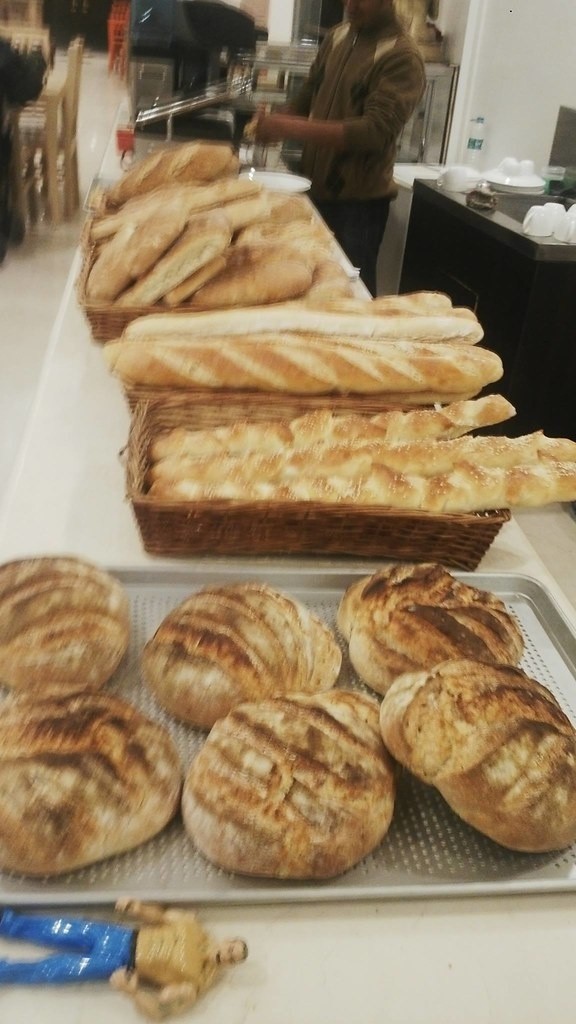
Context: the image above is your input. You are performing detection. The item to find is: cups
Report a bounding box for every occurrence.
[436,168,468,193]
[489,156,542,185]
[522,201,576,242]
[540,164,566,193]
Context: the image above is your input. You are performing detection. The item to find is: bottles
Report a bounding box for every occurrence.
[464,117,486,168]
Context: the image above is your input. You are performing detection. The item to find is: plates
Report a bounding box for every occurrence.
[237,170,312,193]
[484,174,546,196]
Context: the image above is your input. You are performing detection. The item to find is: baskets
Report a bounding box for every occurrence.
[120,385,512,573]
[77,191,303,340]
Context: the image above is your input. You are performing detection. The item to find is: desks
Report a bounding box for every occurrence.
[1,97,574,1024]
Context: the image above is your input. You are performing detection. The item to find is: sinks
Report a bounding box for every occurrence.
[455,190,576,228]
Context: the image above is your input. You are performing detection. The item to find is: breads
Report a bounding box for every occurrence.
[103,291,503,405]
[88,141,356,309]
[146,394,576,513]
[0,557,576,881]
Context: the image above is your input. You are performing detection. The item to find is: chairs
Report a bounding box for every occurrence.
[107,3,129,76]
[0,24,82,222]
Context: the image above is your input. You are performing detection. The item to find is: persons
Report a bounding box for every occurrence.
[248,1,426,301]
[0,35,47,265]
[1,897,249,1023]
